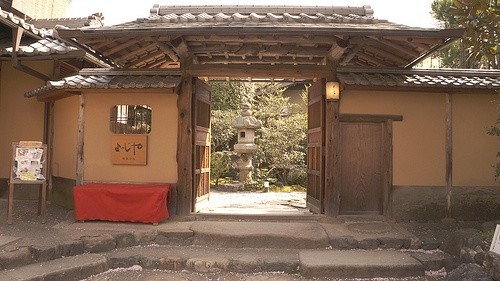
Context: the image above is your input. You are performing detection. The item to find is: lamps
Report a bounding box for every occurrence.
[326,77,340,102]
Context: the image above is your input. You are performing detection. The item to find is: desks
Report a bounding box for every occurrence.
[74,182,174,226]
